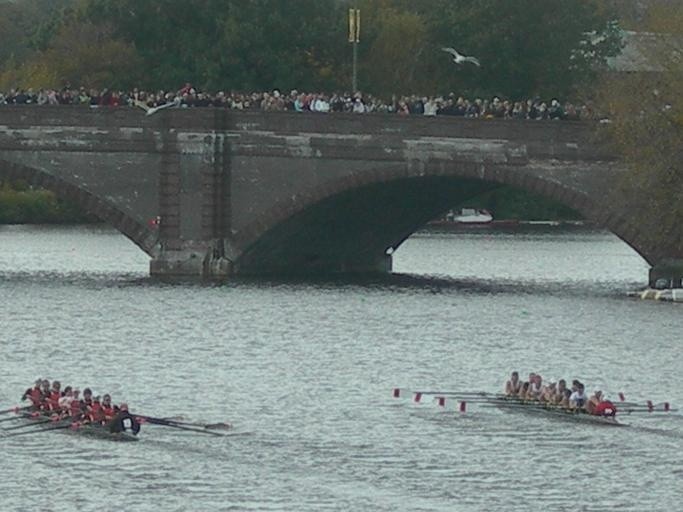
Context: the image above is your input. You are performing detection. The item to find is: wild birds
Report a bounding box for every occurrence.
[442,47,480,66]
[130,98,179,117]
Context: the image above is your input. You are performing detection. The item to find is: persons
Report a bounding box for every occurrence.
[505,371,603,414]
[1,81,600,120]
[22,377,127,433]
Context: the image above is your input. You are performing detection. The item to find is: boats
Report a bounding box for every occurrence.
[13,406,140,442]
[423,205,520,231]
[487,396,632,428]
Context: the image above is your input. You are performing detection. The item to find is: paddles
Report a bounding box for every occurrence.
[603,392,682,419]
[411,391,571,411]
[134,414,231,436]
